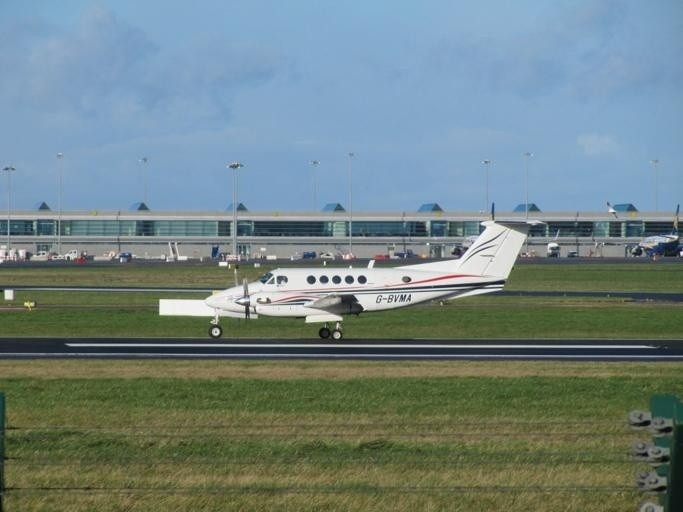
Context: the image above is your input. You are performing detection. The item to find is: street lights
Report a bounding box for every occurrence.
[138,157,150,203]
[649,159,657,211]
[55,151,63,255]
[343,149,354,251]
[481,159,493,211]
[523,150,534,217]
[226,161,244,254]
[308,160,320,212]
[2,165,17,256]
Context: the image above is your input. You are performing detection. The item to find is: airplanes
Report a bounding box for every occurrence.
[603,203,683,259]
[205,220,546,341]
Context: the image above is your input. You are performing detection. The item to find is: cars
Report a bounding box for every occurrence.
[34,249,78,261]
